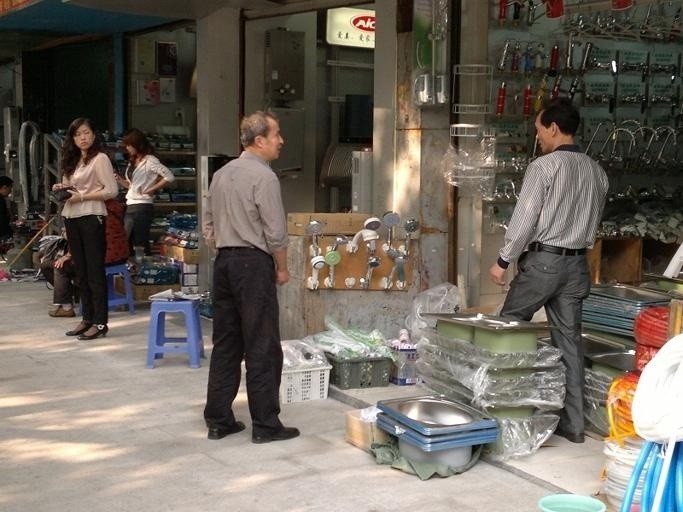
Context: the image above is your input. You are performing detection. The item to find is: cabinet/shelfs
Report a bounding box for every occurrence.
[44,134,198,252]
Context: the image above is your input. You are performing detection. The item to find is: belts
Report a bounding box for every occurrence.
[528,241,586,257]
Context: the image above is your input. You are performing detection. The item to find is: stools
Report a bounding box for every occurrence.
[146,298,204,369]
[79,265,134,316]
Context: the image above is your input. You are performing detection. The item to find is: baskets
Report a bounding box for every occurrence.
[390,348,425,385]
[279,362,333,403]
[326,352,393,389]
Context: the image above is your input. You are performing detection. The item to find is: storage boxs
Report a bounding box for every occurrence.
[345,406,390,454]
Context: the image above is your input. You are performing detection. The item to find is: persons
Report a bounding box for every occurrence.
[202,111,301,442]
[114,128,175,256]
[488,97,609,443]
[52,117,118,340]
[0,175,15,255]
[48,199,129,316]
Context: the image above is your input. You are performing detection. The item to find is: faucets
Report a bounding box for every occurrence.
[496,0,683,171]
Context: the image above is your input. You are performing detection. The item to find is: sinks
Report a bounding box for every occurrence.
[374,281,682,466]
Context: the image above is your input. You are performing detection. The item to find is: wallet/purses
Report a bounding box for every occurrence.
[52,185,77,201]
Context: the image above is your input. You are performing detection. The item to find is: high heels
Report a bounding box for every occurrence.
[66,320,108,340]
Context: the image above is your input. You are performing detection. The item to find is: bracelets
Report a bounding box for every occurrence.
[81,194,84,202]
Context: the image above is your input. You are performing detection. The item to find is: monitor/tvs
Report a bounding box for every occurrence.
[317,7,375,52]
[338,94,372,142]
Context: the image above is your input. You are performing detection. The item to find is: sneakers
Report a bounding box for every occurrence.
[50,305,76,317]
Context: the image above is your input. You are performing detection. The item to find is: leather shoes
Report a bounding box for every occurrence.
[209,422,245,440]
[252,427,299,442]
